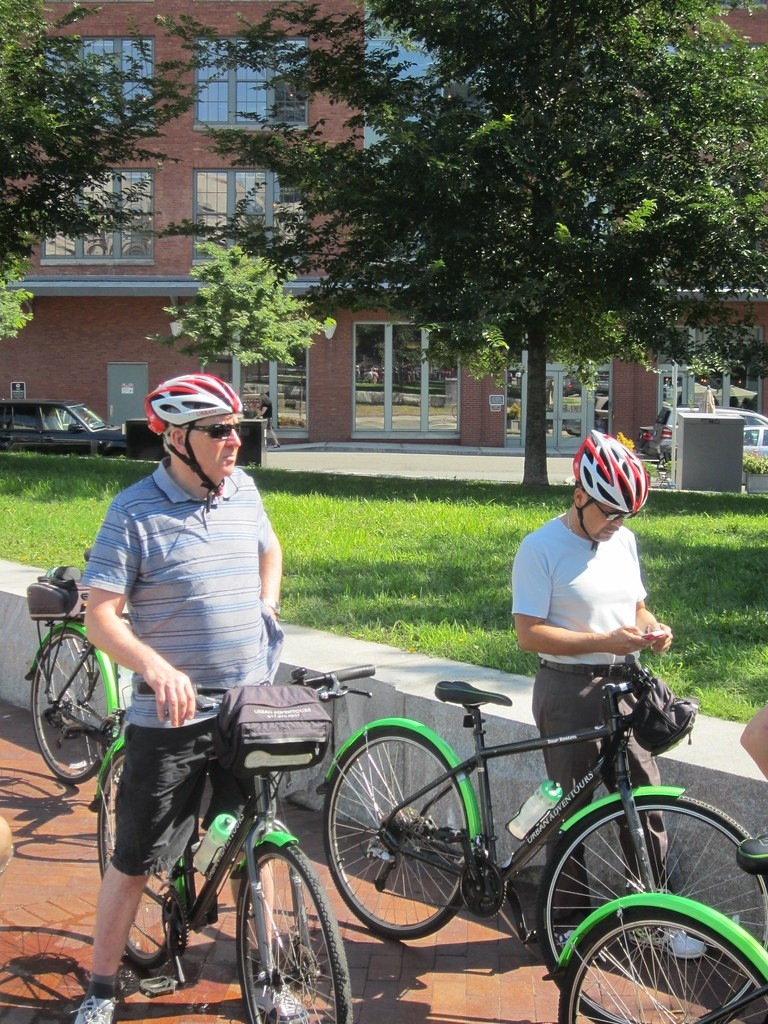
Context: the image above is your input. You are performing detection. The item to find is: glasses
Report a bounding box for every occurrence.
[580,487,638,520]
[179,423,241,440]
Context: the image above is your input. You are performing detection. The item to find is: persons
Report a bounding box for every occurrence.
[71,370,284,1024]
[256,391,281,449]
[740,702,768,781]
[356,363,416,384]
[512,429,708,958]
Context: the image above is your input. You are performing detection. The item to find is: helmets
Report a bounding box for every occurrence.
[573,429,649,514]
[145,374,243,434]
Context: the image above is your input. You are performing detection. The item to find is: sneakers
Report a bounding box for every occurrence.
[253,980,309,1024]
[552,929,597,958]
[628,926,707,958]
[70,997,115,1024]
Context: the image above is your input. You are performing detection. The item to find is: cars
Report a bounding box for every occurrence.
[0,398,127,457]
[562,378,581,397]
[652,403,768,462]
[284,381,306,401]
[566,385,609,436]
[596,374,609,395]
[635,427,656,456]
[743,425,768,455]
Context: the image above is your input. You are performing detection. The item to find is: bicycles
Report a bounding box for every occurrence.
[88,664,376,1024]
[317,654,768,1023]
[542,833,767,1023]
[24,548,125,784]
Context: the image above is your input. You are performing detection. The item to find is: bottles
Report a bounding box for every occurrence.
[194,813,237,872]
[509,779,562,839]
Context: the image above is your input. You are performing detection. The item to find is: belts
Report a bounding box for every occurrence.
[540,659,634,680]
